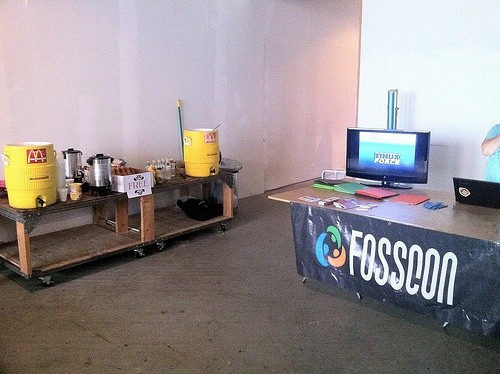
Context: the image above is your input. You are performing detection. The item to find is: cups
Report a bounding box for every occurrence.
[65,179,74,194]
[69,182,82,201]
[58,188,68,201]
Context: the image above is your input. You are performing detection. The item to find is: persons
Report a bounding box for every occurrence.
[481,124,499,183]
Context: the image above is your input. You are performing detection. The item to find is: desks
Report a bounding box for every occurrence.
[267,178,500,340]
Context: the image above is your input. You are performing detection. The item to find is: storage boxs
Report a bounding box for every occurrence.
[111,171,154,193]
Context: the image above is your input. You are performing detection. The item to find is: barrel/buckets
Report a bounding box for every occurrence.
[183,128,219,177]
[87,153,114,195]
[61,148,83,183]
[0,141,57,209]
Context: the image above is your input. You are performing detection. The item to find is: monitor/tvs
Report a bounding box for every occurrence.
[347,127,431,190]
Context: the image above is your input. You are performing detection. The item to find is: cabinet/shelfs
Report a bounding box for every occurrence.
[0,166,239,288]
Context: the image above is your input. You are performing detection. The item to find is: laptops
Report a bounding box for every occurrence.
[452,177,500,210]
